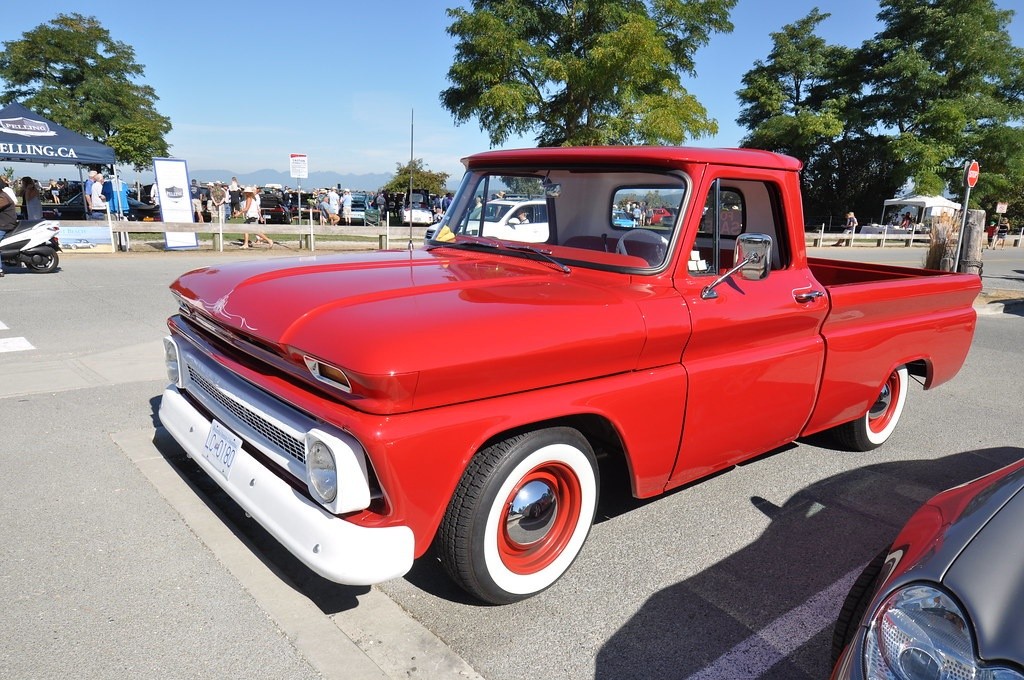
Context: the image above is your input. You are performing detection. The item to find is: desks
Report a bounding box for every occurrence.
[859,225,921,235]
[297,208,322,225]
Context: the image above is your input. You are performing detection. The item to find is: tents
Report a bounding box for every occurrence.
[881,187,961,227]
[0,100,131,251]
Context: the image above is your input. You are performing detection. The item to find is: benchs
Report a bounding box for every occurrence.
[293,215,302,224]
[563,236,737,275]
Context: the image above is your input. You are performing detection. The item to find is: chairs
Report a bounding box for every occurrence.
[364,209,380,227]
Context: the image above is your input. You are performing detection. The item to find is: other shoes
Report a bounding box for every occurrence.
[270,240,273,248]
[239,245,248,249]
[0,272,4,277]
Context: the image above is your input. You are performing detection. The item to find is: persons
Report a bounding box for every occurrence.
[832,211,858,247]
[986,218,1010,250]
[890,212,912,229]
[0,168,672,277]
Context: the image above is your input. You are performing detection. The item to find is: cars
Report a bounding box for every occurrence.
[41,191,161,221]
[646,208,671,225]
[611,211,639,228]
[42,180,370,225]
[424,195,549,246]
[827,458,1024,680]
[398,188,433,226]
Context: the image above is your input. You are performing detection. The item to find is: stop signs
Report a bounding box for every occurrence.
[967,161,979,188]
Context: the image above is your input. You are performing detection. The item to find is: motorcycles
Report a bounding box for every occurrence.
[0,220,62,273]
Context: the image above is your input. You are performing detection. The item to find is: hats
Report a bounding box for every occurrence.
[221,183,228,186]
[192,179,196,182]
[243,187,253,192]
[215,181,221,184]
[331,187,337,191]
[344,189,349,192]
[850,212,854,216]
[206,182,214,186]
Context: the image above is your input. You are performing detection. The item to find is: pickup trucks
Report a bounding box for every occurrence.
[155,146,984,607]
